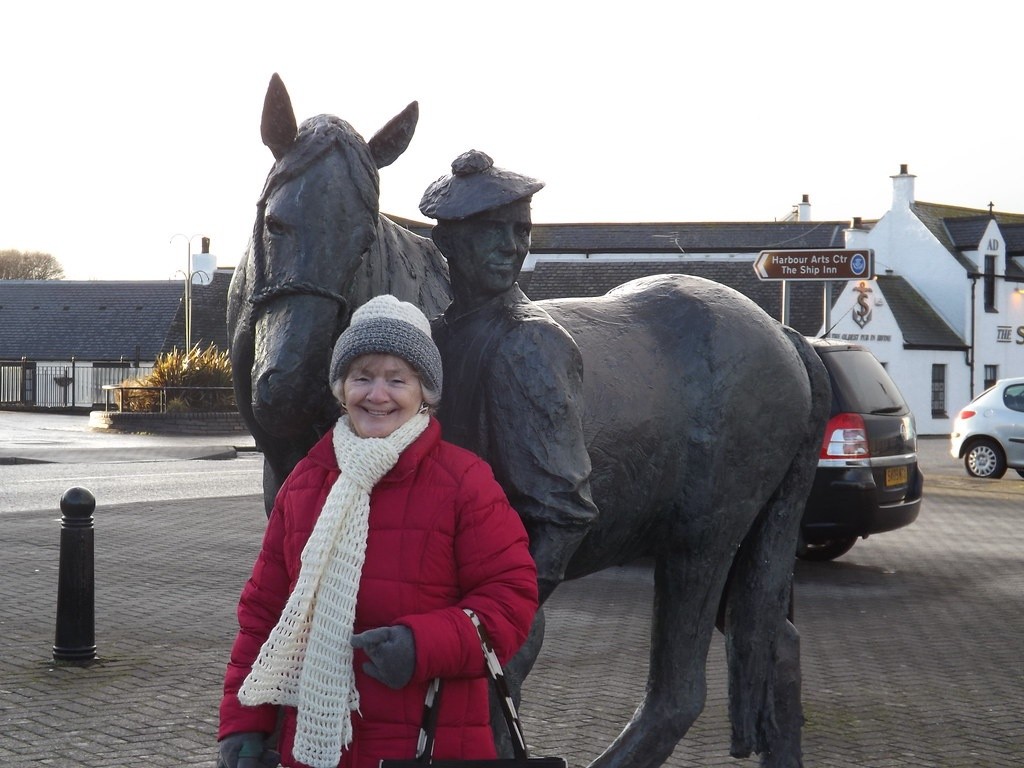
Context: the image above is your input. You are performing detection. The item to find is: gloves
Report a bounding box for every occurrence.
[351,626,415,690]
[217,731,281,768]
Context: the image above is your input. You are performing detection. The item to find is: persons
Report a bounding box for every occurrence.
[216,294,539,768]
[420,149,600,708]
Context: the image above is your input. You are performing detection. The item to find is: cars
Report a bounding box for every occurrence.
[950,377,1024,479]
[795,336,924,560]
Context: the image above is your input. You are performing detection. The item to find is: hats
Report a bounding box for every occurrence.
[329,294,443,396]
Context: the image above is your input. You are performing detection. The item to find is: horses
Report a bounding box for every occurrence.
[227,71,832,768]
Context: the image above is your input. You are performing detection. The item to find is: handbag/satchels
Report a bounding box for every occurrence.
[378,610,568,768]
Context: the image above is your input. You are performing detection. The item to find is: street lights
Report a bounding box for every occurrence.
[168,234,210,363]
